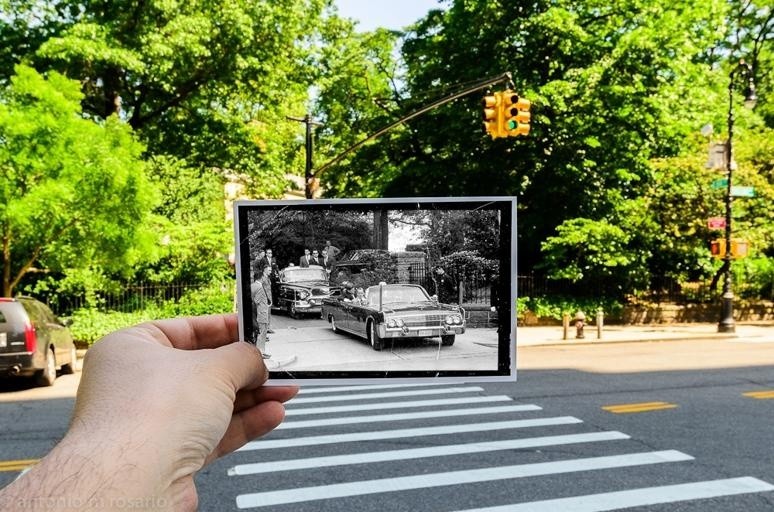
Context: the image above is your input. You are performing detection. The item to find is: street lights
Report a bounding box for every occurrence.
[716,61,753,334]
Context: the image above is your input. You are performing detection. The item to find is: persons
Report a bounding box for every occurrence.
[251,241,387,359]
[0,313,300,512]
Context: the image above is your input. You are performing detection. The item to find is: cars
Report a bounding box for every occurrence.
[319,282,469,351]
[0,294,78,388]
[270,265,342,321]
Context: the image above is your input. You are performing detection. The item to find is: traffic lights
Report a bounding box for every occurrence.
[482,89,531,140]
[730,238,751,260]
[709,237,727,259]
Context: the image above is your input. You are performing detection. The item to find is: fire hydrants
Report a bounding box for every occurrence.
[574,311,588,339]
